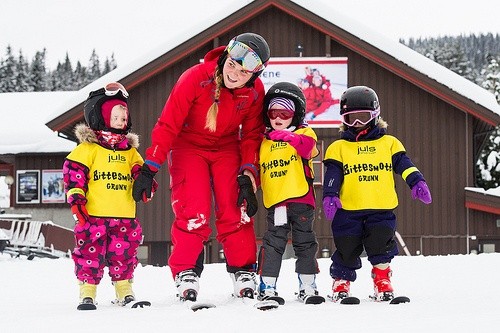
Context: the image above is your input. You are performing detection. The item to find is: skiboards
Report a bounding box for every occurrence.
[191,300,278,312]
[265,296,326,304]
[76,301,152,311]
[340,296,410,305]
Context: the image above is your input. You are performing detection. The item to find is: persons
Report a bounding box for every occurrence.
[256,82,328,305]
[63,83,157,308]
[133,32,269,302]
[297,66,332,121]
[49,177,64,197]
[321,86,432,304]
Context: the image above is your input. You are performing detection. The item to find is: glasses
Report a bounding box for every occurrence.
[267,107,295,120]
[104,82,130,96]
[342,110,375,127]
[230,40,264,72]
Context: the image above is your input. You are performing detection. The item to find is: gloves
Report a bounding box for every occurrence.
[411,182,432,204]
[71,204,88,226]
[236,175,258,218]
[132,162,156,202]
[323,196,341,222]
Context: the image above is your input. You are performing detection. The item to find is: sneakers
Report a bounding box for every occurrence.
[259,274,284,304]
[80,283,96,301]
[114,279,134,301]
[372,265,393,299]
[235,271,256,296]
[297,274,320,300]
[176,271,198,301]
[332,278,350,300]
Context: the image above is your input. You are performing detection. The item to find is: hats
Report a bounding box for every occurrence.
[268,97,296,111]
[100,99,129,130]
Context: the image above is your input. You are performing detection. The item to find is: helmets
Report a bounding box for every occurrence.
[83,88,132,131]
[236,33,270,64]
[263,81,305,127]
[340,85,379,116]
